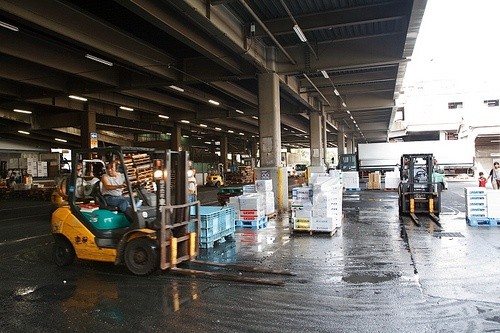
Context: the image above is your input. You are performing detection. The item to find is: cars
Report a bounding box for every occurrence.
[291,163,308,176]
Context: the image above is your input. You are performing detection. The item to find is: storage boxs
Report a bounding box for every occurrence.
[6,152,48,192]
[367,171,381,189]
[466,187,499,218]
[342,171,359,189]
[384,171,402,188]
[228,179,275,221]
[189,205,236,249]
[291,170,343,232]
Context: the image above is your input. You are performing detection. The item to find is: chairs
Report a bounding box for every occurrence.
[84,176,120,211]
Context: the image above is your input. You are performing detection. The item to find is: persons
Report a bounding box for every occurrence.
[76,163,87,197]
[394,164,399,171]
[101,161,141,220]
[478,162,500,190]
